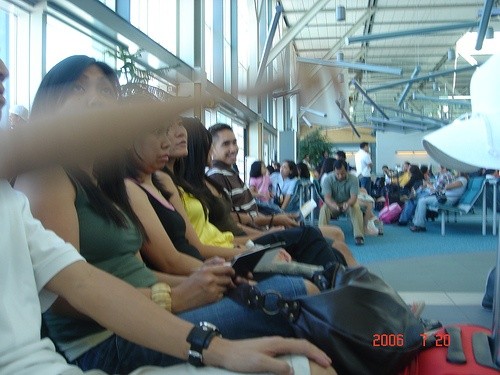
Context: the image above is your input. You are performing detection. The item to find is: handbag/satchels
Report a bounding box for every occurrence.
[379,203,402,224]
[434,191,447,203]
[226,261,424,375]
[274,183,284,208]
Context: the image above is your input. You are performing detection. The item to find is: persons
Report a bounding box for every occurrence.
[318,158,364,246]
[355,141,374,196]
[0,54,457,375]
[410,167,468,235]
[480,168,497,180]
[481,267,497,308]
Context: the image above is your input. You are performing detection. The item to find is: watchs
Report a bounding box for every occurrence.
[186,321,223,369]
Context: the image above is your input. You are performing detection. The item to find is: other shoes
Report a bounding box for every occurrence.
[410,225,427,232]
[355,237,364,245]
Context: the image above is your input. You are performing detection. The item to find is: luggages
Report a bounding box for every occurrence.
[404,324,500,375]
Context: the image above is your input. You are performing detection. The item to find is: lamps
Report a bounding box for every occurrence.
[336,0,345,83]
[485,27,494,39]
[447,47,454,61]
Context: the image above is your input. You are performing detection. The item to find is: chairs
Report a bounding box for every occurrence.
[286,179,322,227]
[426,176,499,236]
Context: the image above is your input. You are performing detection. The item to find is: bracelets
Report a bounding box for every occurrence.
[152,283,172,314]
